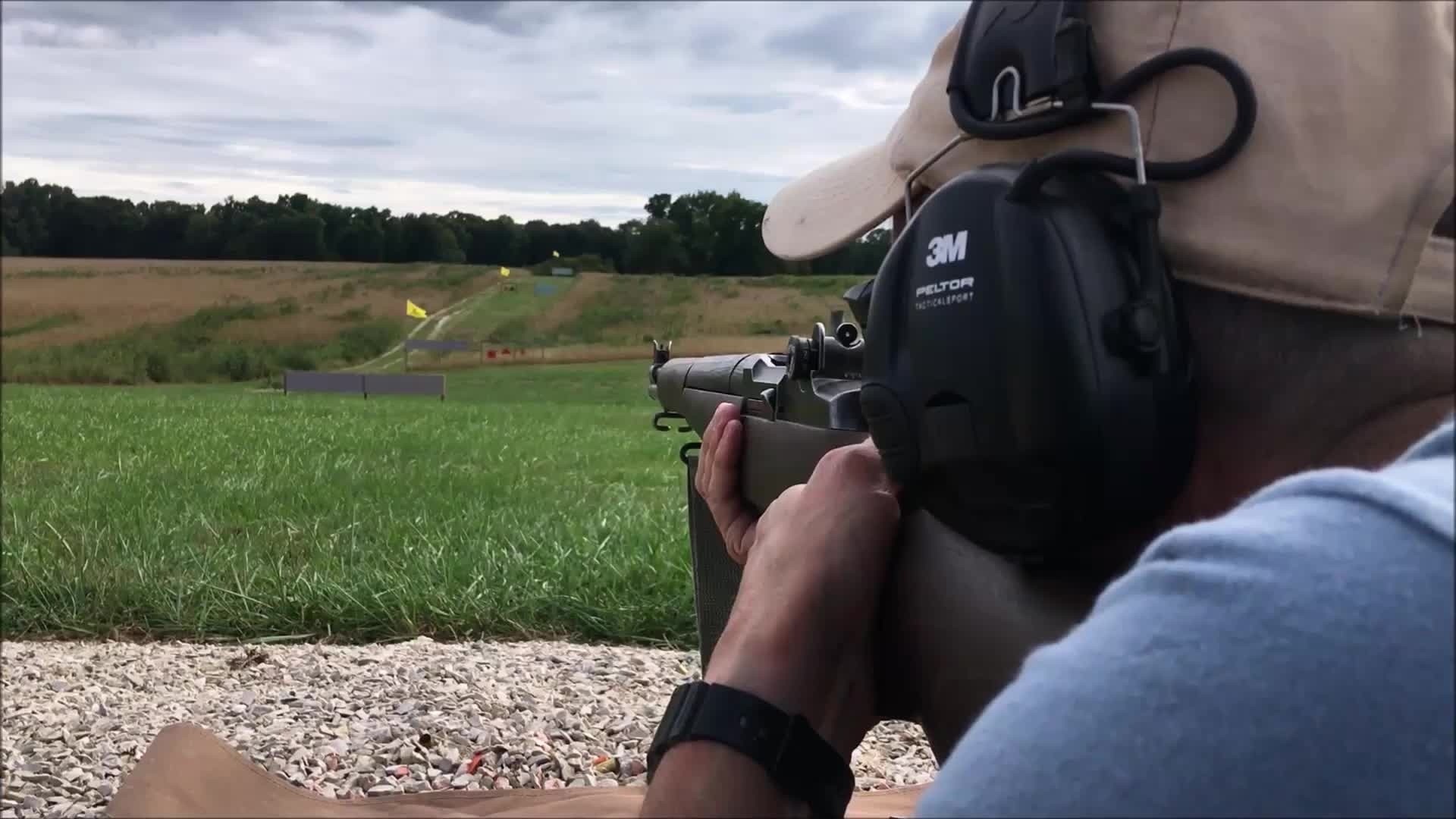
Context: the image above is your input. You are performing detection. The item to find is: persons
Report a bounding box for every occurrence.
[642,1,1456,819]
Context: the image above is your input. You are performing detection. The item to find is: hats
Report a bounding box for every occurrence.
[763,0,1456,322]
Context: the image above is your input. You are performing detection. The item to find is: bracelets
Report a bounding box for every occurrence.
[640,678,856,819]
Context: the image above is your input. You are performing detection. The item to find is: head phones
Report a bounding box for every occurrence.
[859,0,1256,583]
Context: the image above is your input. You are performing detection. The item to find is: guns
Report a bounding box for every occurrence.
[649,322,868,511]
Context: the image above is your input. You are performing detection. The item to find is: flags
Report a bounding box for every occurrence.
[406,299,428,318]
[500,266,510,276]
